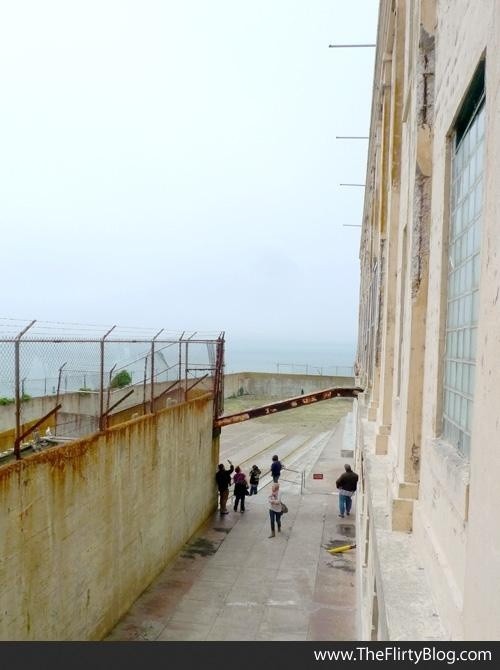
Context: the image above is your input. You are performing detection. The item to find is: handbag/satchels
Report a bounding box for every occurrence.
[281,504,288,513]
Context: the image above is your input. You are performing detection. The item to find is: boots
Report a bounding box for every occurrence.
[268,531,275,538]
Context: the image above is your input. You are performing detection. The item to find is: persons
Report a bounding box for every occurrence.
[335,464,358,518]
[249,465,261,495]
[215,459,234,513]
[267,483,282,538]
[271,455,281,483]
[233,466,249,512]
